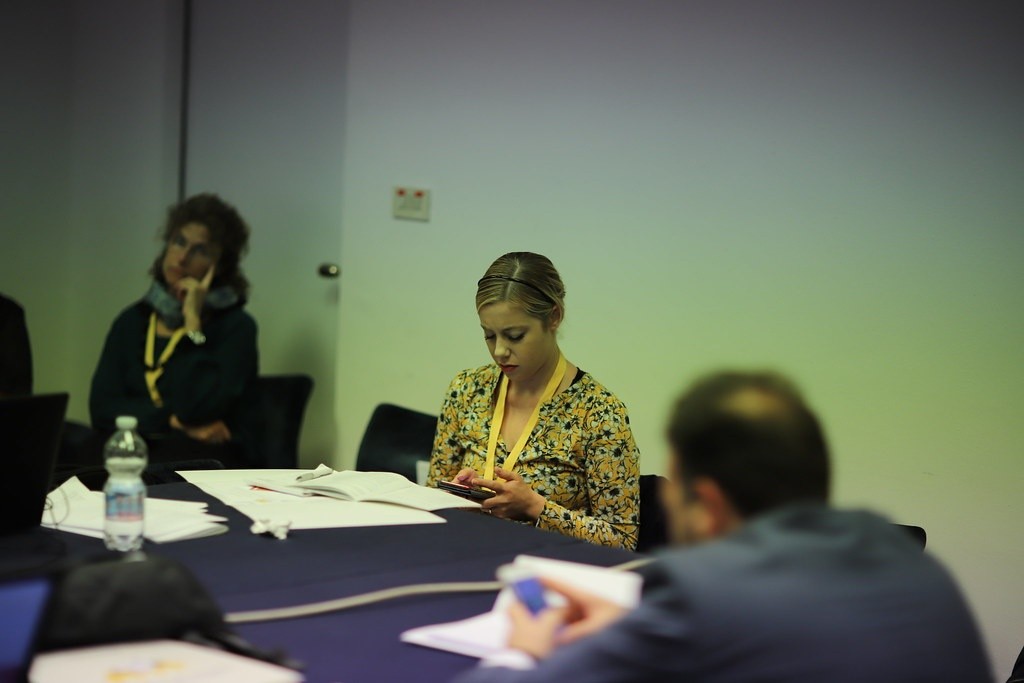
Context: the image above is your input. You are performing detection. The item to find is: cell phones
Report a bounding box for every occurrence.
[439,480,482,492]
[497,563,551,619]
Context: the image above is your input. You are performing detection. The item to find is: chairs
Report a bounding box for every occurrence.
[354,403,438,484]
[235,371,314,470]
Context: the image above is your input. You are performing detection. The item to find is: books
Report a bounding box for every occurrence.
[251,464,484,511]
[400,553,645,660]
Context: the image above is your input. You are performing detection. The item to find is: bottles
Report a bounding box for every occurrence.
[102,415,146,551]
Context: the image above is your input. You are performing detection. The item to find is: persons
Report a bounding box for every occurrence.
[459,371,998,683]
[426,249,640,556]
[90,192,254,469]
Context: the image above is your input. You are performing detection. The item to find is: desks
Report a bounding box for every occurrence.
[0,481,659,683]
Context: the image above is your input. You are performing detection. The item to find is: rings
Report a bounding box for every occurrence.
[489,509,492,515]
